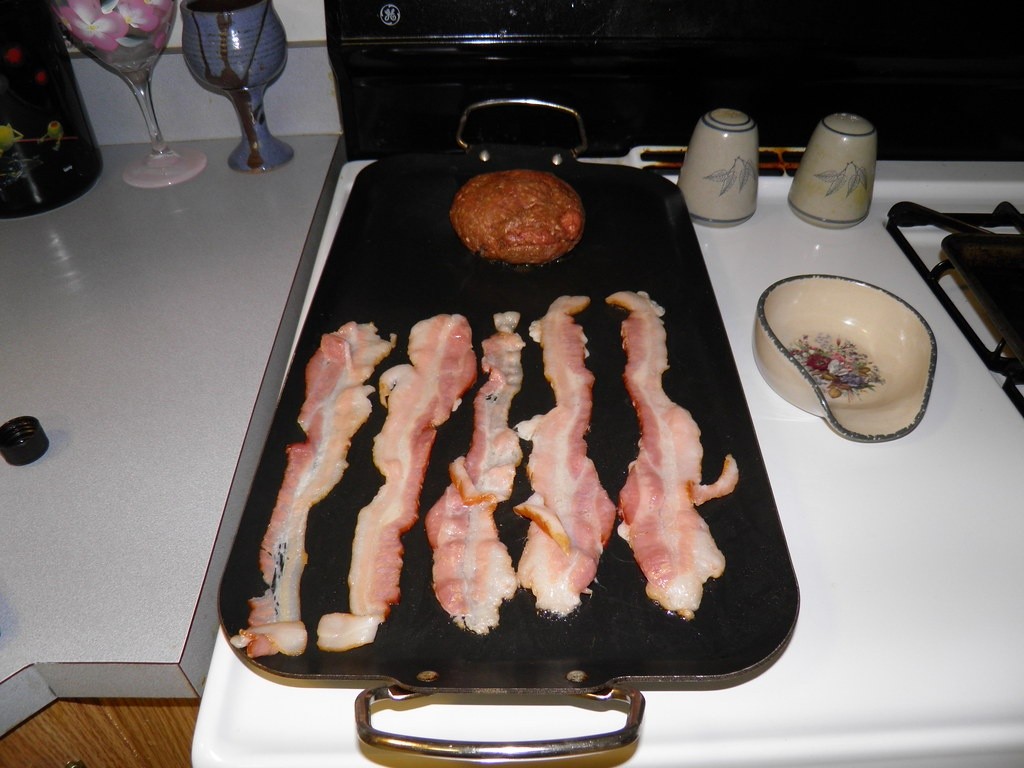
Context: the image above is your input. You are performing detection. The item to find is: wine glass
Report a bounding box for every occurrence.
[49,0,211,188]
[178,0,296,172]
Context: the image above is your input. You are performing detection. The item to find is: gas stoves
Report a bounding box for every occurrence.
[184,0,1024,763]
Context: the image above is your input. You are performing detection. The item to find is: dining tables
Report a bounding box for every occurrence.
[189,147,1023,768]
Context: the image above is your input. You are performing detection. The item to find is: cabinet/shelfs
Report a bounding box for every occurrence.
[0,133,343,768]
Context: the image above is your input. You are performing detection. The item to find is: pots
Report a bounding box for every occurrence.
[210,95,806,765]
